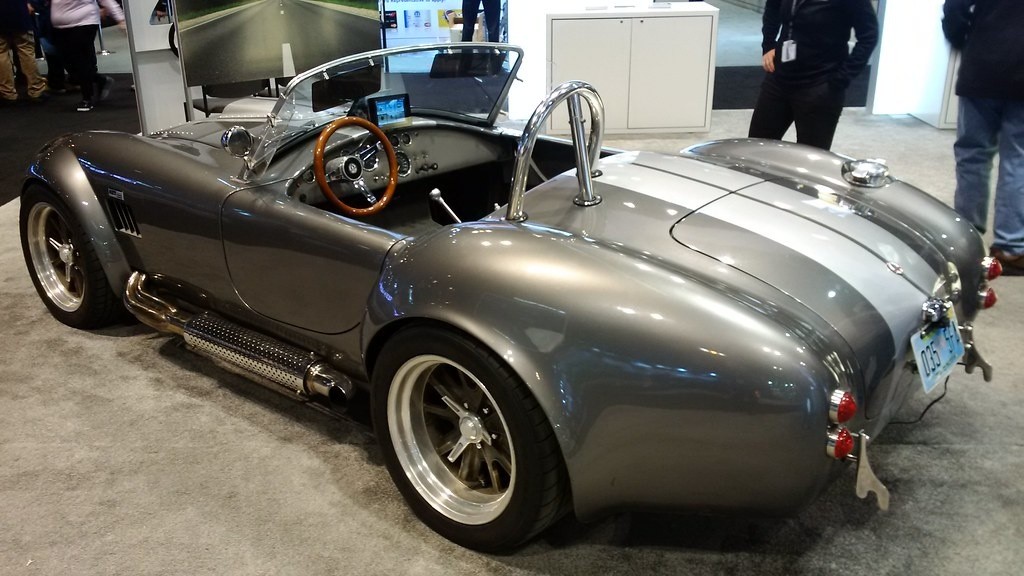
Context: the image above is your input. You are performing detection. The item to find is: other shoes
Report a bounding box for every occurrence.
[28,77,47,98]
[66,82,81,89]
[77,98,94,112]
[56,85,66,92]
[990,244,1024,277]
[100,76,114,101]
[4,89,18,100]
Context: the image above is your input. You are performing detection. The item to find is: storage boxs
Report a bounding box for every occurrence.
[449,11,487,53]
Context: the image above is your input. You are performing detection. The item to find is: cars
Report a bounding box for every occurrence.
[19,41,1004,555]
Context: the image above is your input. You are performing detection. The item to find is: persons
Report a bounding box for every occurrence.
[462,0,500,53]
[132,0,168,90]
[942,0,1024,276]
[50,0,127,112]
[0,0,81,102]
[748,0,879,151]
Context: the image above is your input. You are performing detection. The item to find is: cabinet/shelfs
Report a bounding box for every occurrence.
[546,2,719,138]
